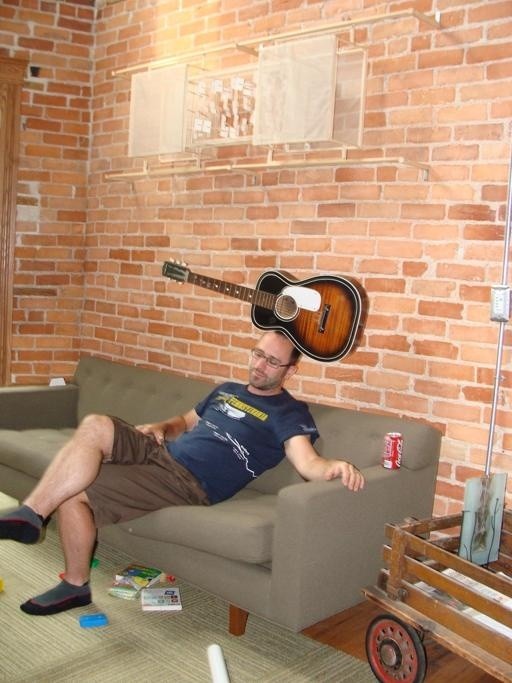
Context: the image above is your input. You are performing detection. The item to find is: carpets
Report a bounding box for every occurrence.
[0,492,379,683]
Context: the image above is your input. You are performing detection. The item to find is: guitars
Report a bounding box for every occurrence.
[162,258,369,362]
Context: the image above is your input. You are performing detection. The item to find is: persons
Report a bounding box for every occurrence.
[0,327,367,617]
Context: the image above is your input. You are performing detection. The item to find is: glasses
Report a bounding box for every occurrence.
[251,347,295,369]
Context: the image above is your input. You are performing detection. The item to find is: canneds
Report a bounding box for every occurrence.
[380,432,403,469]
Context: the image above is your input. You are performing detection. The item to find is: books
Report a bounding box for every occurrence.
[140,586,183,611]
[115,560,162,588]
[108,579,144,601]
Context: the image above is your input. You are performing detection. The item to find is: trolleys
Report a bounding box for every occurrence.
[357,500,511,683]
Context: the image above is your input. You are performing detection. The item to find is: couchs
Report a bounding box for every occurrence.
[0,356,441,634]
